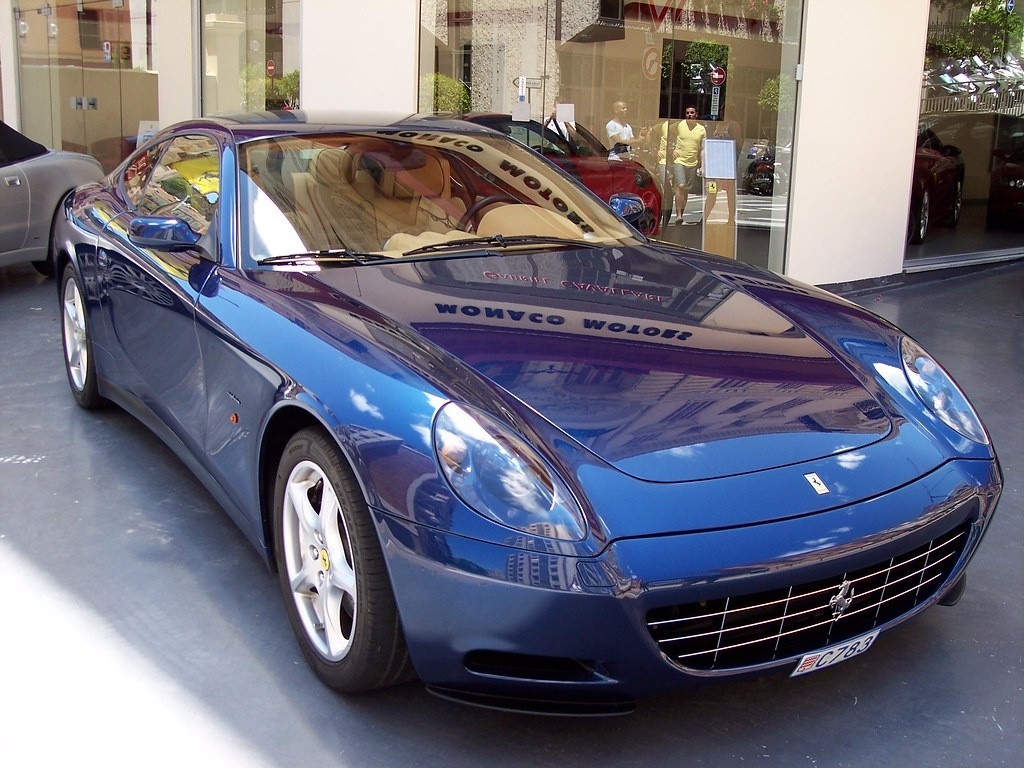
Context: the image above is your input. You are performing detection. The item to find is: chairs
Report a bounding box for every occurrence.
[313,142,475,256]
[249,151,344,259]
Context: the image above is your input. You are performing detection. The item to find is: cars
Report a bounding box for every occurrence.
[742,114,966,246]
[994,132,1023,225]
[340,109,664,242]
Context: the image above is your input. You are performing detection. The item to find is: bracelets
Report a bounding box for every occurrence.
[546,119,549,122]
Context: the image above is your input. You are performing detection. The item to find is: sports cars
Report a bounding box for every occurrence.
[0,121,108,277]
[54,115,1004,717]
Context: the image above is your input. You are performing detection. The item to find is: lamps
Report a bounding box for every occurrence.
[48,22,59,41]
[19,18,29,39]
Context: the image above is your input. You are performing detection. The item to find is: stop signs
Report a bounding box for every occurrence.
[711,67,726,85]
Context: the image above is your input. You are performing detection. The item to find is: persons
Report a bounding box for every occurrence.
[605,99,645,161]
[541,97,577,155]
[647,105,707,225]
[693,102,742,227]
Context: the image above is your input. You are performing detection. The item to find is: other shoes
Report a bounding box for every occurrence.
[675,217,684,226]
